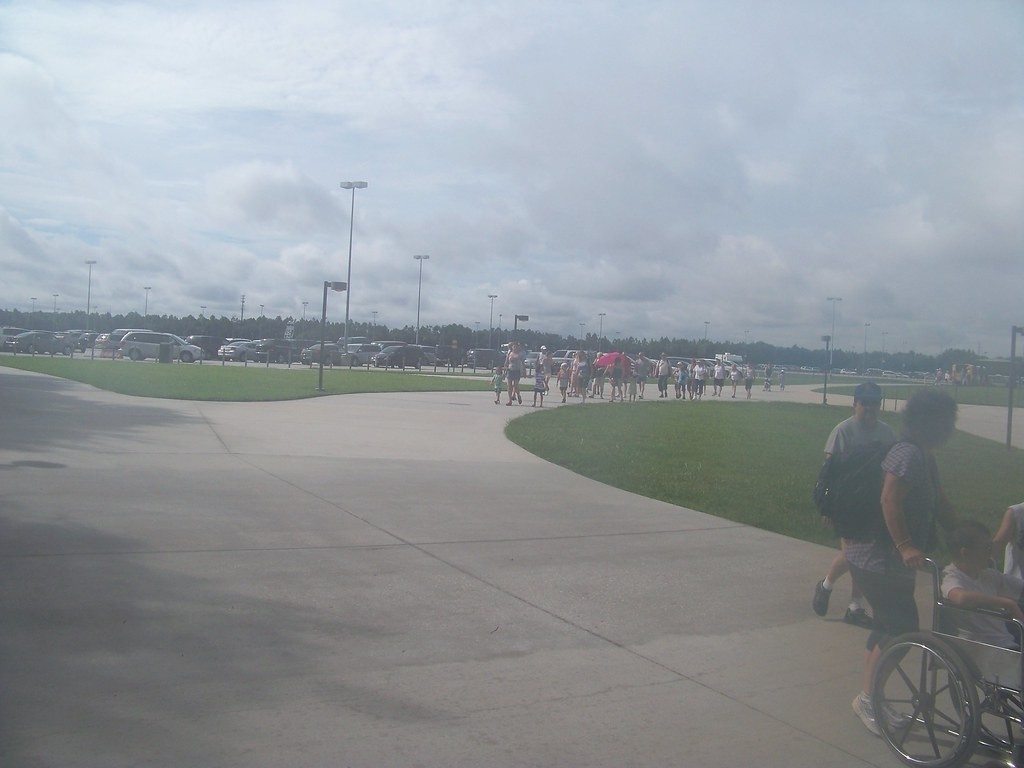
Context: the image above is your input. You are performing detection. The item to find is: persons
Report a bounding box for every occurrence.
[491,339,786,407]
[934,360,989,385]
[940,520,1024,652]
[842,389,958,740]
[991,503,1023,583]
[812,382,899,629]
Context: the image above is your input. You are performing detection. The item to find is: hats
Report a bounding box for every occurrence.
[854,383,881,401]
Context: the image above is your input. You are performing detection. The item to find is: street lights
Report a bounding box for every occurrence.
[413,253,432,347]
[1005,323,1024,445]
[142,282,152,312]
[821,333,834,410]
[487,293,499,350]
[513,314,530,343]
[85,259,101,317]
[315,281,347,392]
[339,180,369,280]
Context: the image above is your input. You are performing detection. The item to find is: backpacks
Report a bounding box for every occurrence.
[812,442,887,540]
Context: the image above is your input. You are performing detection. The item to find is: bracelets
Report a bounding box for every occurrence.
[894,536,913,550]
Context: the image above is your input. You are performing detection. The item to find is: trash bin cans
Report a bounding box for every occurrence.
[159,342,173,364]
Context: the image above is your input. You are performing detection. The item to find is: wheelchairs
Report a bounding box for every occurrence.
[864,551,1023,766]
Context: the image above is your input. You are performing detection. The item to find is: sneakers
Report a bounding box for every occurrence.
[843,608,875,629]
[880,695,906,728]
[852,690,884,737]
[812,577,832,616]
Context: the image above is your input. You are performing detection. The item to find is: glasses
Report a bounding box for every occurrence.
[862,400,881,407]
[971,541,995,552]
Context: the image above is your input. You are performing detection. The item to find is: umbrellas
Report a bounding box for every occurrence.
[597,351,626,367]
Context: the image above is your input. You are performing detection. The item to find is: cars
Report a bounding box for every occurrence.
[0,327,820,377]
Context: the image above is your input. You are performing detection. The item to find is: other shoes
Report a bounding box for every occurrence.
[485,387,789,412]
